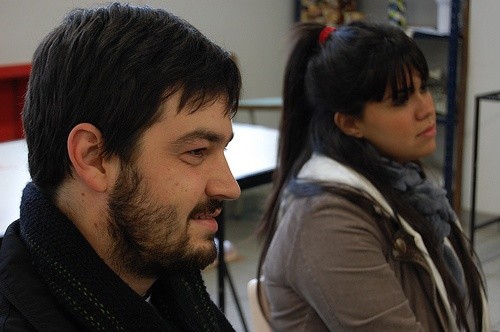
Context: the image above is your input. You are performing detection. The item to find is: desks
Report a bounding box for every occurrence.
[0,122,281,332]
[469,92,500,258]
[232,98,284,126]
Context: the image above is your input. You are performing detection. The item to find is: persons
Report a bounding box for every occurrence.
[0,2,241,331]
[258,18,492,331]
